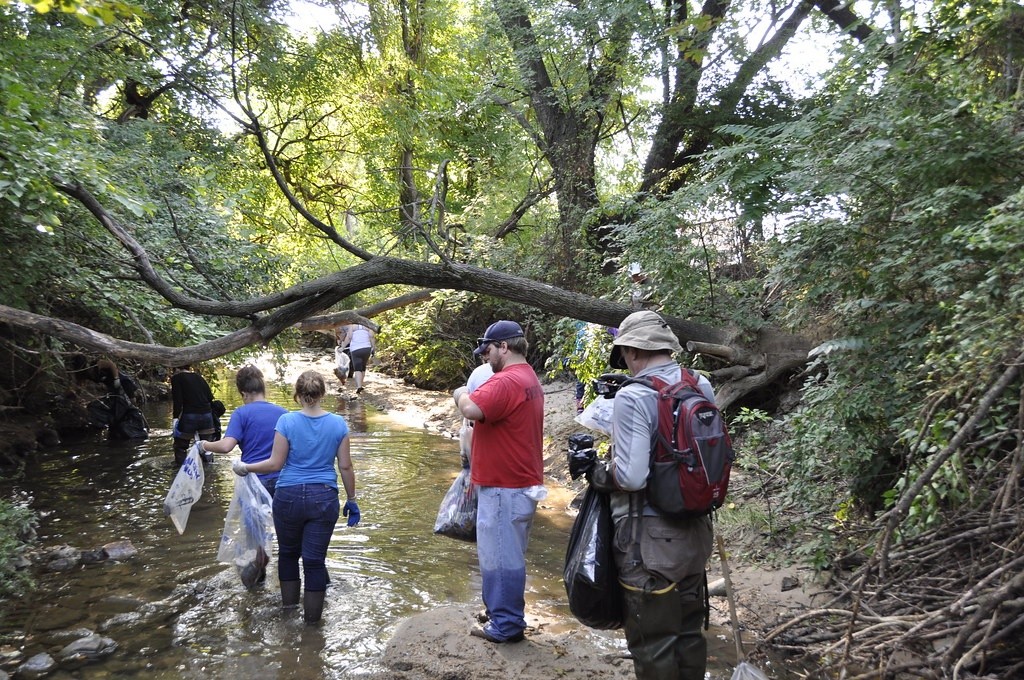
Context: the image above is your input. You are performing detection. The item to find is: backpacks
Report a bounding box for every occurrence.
[620,369,736,518]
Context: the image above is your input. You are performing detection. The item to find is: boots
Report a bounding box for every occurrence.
[280,579,301,604]
[304,591,324,624]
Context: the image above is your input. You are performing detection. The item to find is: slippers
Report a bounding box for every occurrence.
[357,386,363,394]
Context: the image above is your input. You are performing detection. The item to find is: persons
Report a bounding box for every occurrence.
[232,371,361,622]
[170,364,215,462]
[576,321,618,415]
[585,311,714,680]
[337,323,376,393]
[455,321,544,642]
[196,367,288,584]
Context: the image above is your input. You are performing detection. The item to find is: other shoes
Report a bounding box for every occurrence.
[471,609,525,643]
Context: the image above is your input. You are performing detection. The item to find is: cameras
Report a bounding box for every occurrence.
[592,380,617,395]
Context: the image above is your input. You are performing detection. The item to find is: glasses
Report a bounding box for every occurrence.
[476,337,501,347]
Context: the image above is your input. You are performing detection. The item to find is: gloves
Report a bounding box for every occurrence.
[460,452,471,470]
[453,385,470,408]
[197,440,206,454]
[172,419,181,438]
[343,497,361,525]
[232,462,249,477]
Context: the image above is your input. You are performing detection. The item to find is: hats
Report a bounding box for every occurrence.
[473,320,524,354]
[609,309,684,368]
